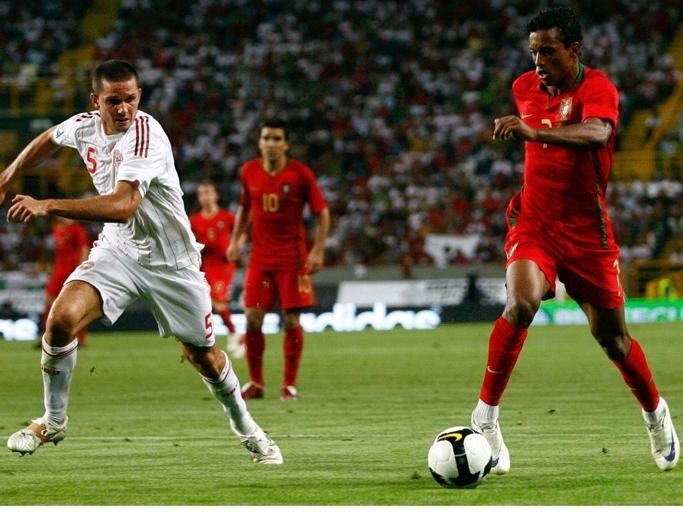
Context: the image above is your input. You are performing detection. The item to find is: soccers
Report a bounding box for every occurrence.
[427,426,494,489]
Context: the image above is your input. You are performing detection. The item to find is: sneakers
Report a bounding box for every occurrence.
[230,419,283,464]
[642,397,680,470]
[280,382,298,400]
[471,409,511,475]
[241,382,265,400]
[7,415,69,456]
[227,335,247,359]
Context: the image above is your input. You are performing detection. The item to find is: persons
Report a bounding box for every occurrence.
[225,118,331,403]
[187,178,248,355]
[0,58,284,466]
[470,6,681,474]
[1,1,683,274]
[33,217,89,350]
